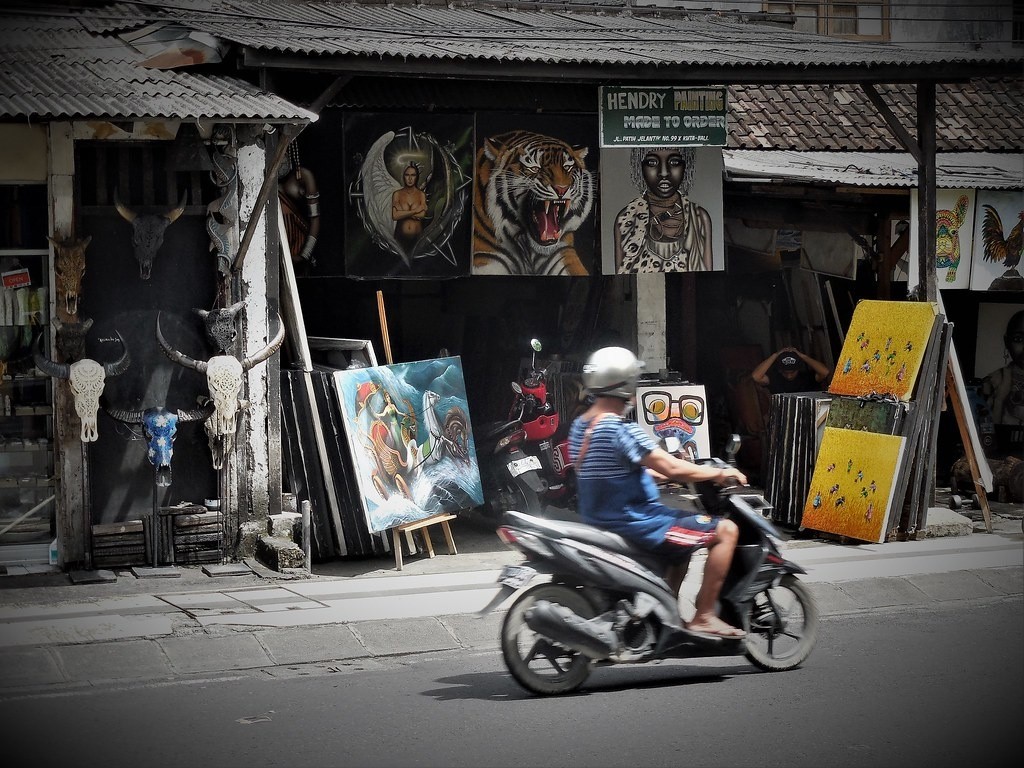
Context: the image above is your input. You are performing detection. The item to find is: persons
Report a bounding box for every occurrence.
[568,347,747,639]
[752,347,830,394]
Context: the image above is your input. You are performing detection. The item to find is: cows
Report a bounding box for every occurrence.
[114,187,188,281]
[34,312,285,488]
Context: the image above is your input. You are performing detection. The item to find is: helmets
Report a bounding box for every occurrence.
[582,347,645,400]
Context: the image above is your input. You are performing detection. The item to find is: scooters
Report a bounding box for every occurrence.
[456,336,584,533]
[471,434,821,696]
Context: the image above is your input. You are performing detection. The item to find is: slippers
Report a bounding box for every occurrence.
[688,626,747,639]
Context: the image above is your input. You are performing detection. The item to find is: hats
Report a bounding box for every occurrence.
[778,351,801,370]
